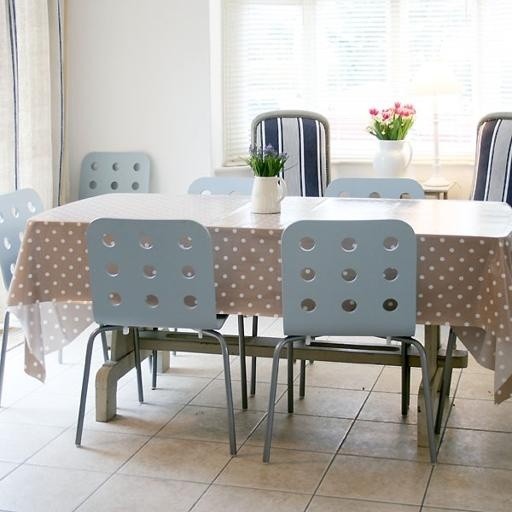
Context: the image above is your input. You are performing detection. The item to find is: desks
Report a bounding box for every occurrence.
[6,193,512,448]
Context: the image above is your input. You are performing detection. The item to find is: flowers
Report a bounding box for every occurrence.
[241,143,290,177]
[364,102,416,140]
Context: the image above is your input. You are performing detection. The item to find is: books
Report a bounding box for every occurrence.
[221,155,250,167]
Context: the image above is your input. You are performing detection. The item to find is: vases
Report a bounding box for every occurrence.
[371,140,413,178]
[250,176,288,214]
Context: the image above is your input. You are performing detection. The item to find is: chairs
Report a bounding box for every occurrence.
[152,176,267,396]
[299,177,436,398]
[79,152,151,199]
[250,110,331,197]
[0,188,109,409]
[75,217,247,456]
[262,219,437,465]
[469,113,512,206]
